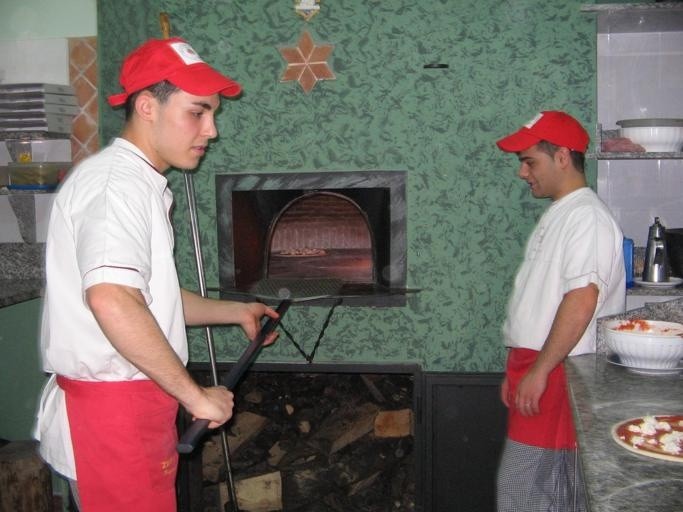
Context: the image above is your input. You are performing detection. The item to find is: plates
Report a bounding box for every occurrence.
[631,276,682,291]
[606,352,682,377]
[616,118,682,128]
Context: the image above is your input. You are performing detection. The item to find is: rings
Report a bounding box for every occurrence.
[525,403,530,407]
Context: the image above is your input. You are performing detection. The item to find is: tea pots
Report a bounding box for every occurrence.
[644,216,671,285]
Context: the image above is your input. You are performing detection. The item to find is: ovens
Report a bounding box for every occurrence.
[214,168,411,310]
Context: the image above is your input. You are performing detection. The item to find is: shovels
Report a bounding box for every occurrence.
[176,277,344,452]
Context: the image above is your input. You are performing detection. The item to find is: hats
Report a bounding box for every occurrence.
[107,36,243,109]
[496,110,590,153]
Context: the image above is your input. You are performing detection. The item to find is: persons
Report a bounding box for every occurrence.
[32,36,279,511]
[494,111,627,512]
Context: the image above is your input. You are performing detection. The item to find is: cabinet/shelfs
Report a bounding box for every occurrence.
[580,0,682,161]
[0,129,72,313]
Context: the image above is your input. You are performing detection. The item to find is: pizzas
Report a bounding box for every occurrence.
[617,415,683,463]
[273,246,326,257]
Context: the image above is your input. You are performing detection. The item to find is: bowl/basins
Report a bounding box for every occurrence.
[600,314,683,370]
[619,127,682,153]
